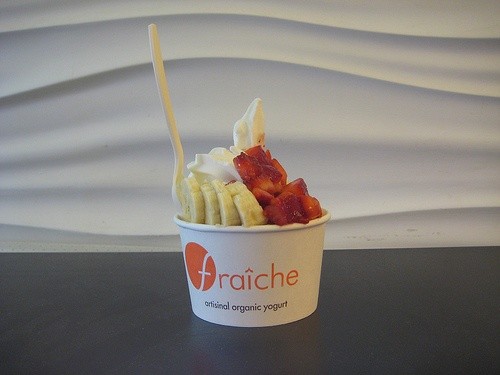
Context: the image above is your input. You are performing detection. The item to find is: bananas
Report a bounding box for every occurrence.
[178,179,268,227]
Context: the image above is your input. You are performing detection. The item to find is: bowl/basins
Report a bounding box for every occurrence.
[172,209,329,327]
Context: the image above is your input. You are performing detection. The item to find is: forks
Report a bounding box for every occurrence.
[148,23,191,221]
